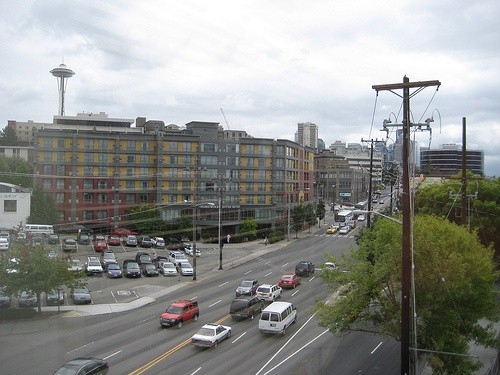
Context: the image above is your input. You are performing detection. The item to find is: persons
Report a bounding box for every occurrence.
[227,232,231,243]
[93,235,96,245]
[209,232,213,240]
[77,234,80,244]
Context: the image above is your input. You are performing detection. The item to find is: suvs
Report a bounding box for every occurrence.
[295,260,315,278]
[62,237,78,252]
[159,299,200,330]
[229,294,267,320]
[255,283,283,303]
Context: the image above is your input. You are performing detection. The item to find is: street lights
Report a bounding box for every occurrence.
[192,201,215,281]
[288,187,310,242]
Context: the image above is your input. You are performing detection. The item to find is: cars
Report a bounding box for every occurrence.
[176,261,193,276]
[0,226,202,279]
[0,290,12,306]
[235,279,260,297]
[70,285,92,305]
[53,356,110,375]
[45,289,65,305]
[18,289,38,307]
[324,261,339,275]
[326,184,403,235]
[191,323,232,348]
[279,274,302,290]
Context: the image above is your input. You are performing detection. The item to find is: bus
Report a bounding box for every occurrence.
[332,209,354,226]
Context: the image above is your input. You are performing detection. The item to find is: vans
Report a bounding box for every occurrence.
[258,301,297,335]
[19,224,55,239]
